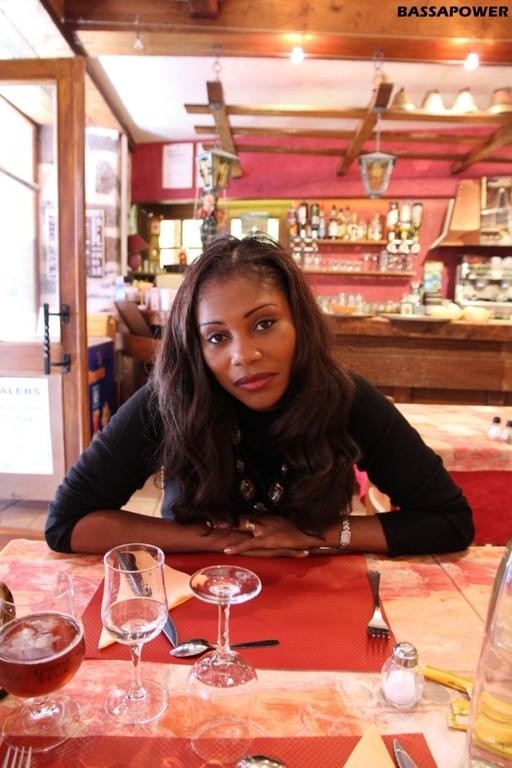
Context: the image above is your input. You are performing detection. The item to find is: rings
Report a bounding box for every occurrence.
[244,518,256,532]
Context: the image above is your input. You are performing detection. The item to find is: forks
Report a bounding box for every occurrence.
[2,745,32,768]
[368,570,388,641]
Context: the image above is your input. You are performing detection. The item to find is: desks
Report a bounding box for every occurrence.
[352,402,512,545]
[0,538,512,768]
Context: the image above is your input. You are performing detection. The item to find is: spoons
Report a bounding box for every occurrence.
[169,632,286,661]
[170,638,279,659]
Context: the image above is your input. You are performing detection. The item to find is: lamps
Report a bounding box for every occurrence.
[195,100,239,197]
[356,107,396,199]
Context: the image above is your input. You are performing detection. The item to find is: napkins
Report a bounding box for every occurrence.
[0,734,441,768]
[78,546,403,672]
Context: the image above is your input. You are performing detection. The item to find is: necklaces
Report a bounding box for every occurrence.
[231,427,288,513]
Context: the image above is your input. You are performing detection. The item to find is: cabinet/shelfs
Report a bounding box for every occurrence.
[290,237,417,278]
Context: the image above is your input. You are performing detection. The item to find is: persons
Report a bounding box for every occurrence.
[37,234,477,560]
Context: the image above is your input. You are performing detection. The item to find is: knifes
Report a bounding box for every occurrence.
[113,540,178,648]
[393,736,415,767]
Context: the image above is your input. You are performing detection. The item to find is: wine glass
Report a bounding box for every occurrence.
[0,568,92,753]
[102,544,171,733]
[188,563,250,766]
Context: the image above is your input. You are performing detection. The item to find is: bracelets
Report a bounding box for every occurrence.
[338,513,352,551]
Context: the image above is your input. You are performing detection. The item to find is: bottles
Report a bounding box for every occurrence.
[461,540,512,768]
[486,416,511,441]
[378,642,423,708]
[289,200,423,315]
